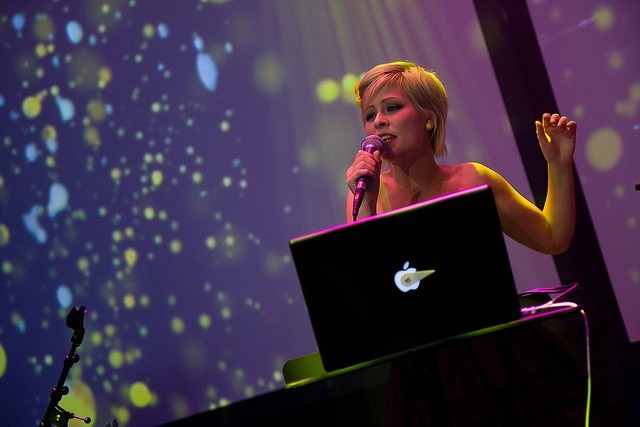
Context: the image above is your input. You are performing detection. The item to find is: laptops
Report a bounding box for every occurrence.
[288,183,523,371]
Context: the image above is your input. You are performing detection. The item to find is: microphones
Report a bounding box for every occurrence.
[351,135,384,219]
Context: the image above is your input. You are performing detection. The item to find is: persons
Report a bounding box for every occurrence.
[345,62,577,256]
